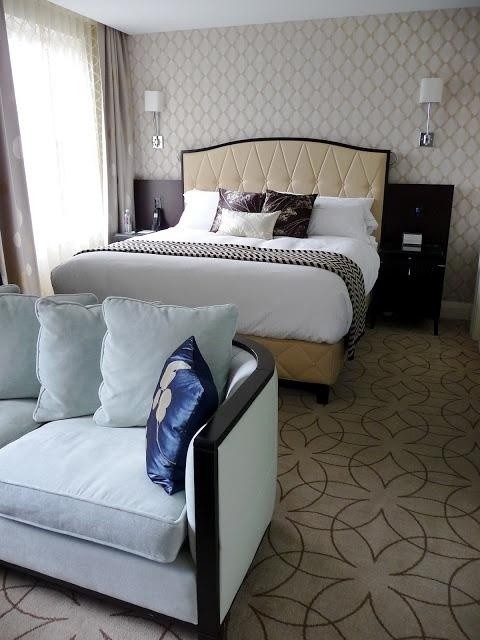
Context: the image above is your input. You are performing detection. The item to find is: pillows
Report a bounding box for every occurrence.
[93,296,240,428]
[146,336,220,494]
[33,296,163,424]
[1,293,101,401]
[175,188,378,241]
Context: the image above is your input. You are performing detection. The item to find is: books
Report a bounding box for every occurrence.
[136,229,155,236]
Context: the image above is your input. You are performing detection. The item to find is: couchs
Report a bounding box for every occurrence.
[0,331,280,639]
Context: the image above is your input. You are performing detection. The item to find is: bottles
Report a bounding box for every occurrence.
[124,208,132,233]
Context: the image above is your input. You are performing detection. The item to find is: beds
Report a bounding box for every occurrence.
[49,136,392,407]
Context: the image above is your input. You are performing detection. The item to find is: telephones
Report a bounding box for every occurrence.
[151,208,162,232]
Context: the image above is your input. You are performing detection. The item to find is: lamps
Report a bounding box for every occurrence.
[144,90,166,149]
[418,77,444,148]
